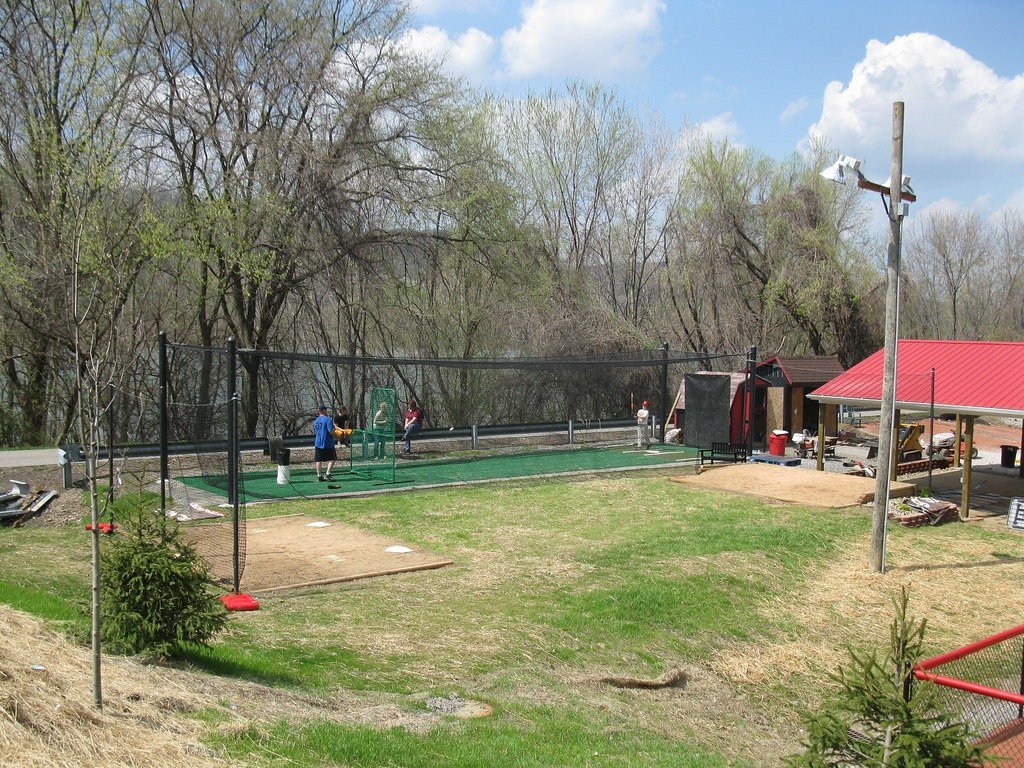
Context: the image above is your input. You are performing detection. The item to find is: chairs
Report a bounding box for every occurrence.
[845,405,862,427]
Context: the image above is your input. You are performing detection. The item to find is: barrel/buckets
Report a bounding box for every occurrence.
[276,465,291,484]
[768,433,787,456]
[999,445,1019,468]
[276,448,291,466]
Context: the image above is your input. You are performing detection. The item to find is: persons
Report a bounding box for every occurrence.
[401,400,422,454]
[635,400,653,451]
[372,402,388,461]
[313,402,351,484]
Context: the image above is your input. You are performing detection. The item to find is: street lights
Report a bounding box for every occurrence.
[818,103,916,573]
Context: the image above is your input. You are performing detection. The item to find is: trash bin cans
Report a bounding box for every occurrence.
[999,445,1020,468]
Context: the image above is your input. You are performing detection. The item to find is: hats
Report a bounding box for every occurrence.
[643,400,650,407]
[319,404,326,410]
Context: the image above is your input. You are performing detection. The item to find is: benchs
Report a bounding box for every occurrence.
[700,443,748,465]
[812,446,835,459]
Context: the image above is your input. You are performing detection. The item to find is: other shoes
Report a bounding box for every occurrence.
[379,455,387,459]
[646,444,652,450]
[373,457,378,461]
[634,447,641,451]
[400,450,409,455]
[324,474,336,481]
[317,476,327,482]
[401,438,407,441]
